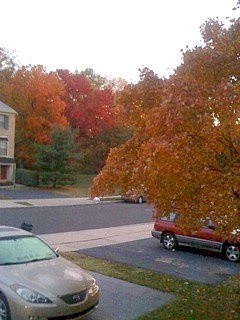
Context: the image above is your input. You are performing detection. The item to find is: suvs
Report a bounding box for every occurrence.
[151,209,240,263]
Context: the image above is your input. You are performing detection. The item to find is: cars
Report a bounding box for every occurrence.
[121,191,148,204]
[0,225,100,320]
